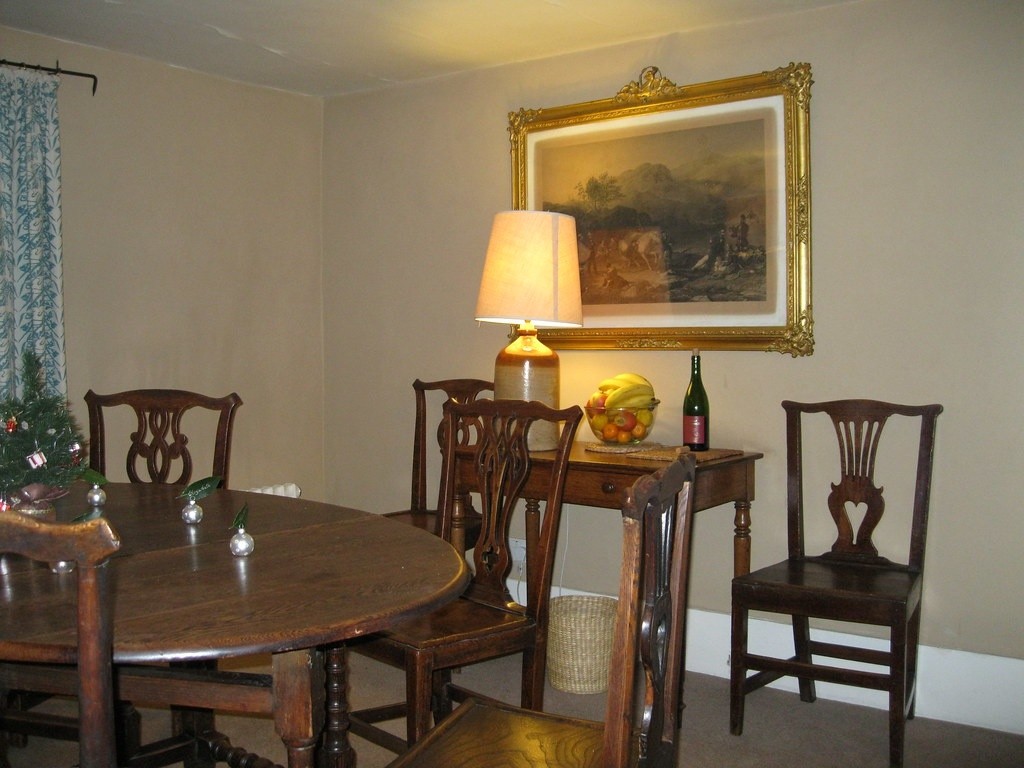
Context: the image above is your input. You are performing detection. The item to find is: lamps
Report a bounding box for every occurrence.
[475,211,584,451]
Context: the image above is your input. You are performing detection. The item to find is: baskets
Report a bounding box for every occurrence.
[545,594,618,695]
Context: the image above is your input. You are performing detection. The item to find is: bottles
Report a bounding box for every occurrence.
[684,356,710,451]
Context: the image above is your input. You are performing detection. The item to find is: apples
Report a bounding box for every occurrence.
[587,391,652,443]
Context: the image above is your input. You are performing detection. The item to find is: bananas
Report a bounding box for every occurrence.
[598,373,659,415]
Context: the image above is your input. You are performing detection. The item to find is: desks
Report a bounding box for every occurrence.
[441,438,764,676]
[0,484,469,768]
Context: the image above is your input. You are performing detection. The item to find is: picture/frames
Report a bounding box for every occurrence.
[506,62,816,359]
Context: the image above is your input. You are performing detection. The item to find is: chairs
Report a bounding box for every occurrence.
[0,509,200,768]
[349,397,584,757]
[381,379,495,551]
[85,389,243,489]
[729,399,944,768]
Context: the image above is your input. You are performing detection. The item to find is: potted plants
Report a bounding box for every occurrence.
[1,350,108,523]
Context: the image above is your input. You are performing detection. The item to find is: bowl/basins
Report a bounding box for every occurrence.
[584,400,659,445]
